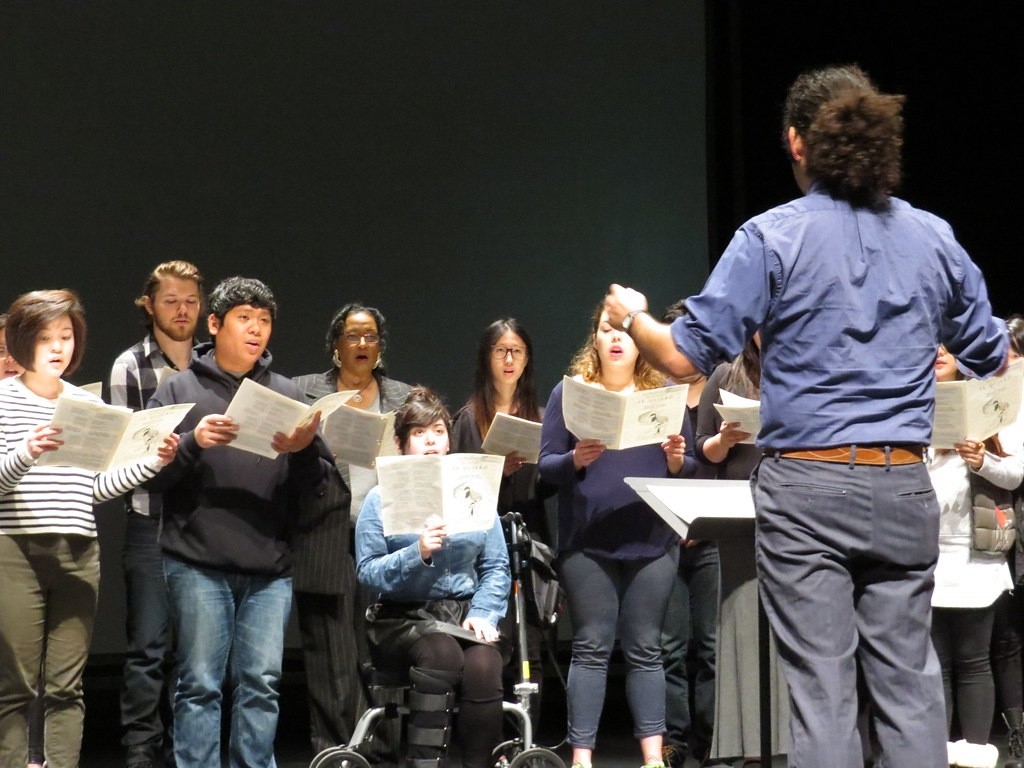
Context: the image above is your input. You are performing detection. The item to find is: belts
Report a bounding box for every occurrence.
[763,445,922,464]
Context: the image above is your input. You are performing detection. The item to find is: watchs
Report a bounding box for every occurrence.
[621,309,647,331]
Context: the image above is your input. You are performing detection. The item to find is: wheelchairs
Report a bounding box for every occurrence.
[308,511,568,768]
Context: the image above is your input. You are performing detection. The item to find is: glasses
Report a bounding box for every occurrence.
[488,345,529,360]
[336,334,381,343]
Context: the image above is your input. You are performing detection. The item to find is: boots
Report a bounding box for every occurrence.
[1001,708,1024,768]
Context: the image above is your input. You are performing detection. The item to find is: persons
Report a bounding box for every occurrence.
[659,299,787,768]
[291,302,415,768]
[145,276,325,768]
[603,69,1011,768]
[451,318,545,740]
[538,299,698,768]
[354,389,512,768]
[110,260,204,768]
[0,289,181,768]
[924,318,1024,768]
[0,313,26,380]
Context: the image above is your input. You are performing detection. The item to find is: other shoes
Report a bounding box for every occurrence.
[661,743,685,767]
